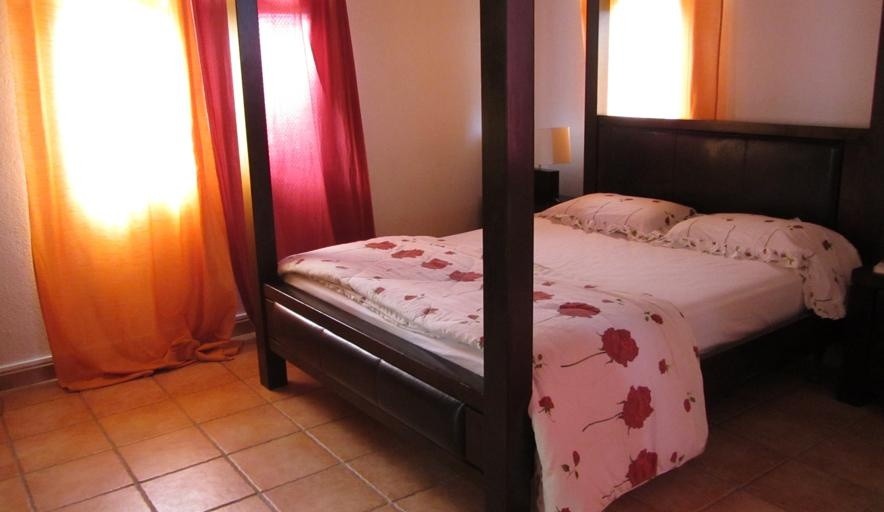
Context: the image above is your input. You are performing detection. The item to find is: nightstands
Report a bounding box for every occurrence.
[838,258,883,409]
[533,195,571,214]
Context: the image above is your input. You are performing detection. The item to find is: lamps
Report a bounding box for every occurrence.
[532,125,572,206]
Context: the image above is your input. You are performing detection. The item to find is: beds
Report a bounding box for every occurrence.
[234,0,884,512]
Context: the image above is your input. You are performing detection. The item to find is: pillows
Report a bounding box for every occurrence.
[673,210,877,321]
[539,174,696,242]
